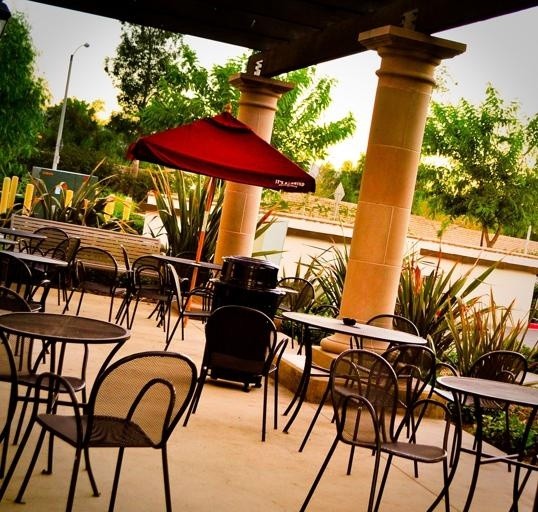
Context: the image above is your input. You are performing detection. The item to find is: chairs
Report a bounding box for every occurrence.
[2,351,198,511]
[2,285,85,446]
[300,349,451,511]
[182,304,289,442]
[331,313,420,438]
[393,349,530,473]
[1,225,316,353]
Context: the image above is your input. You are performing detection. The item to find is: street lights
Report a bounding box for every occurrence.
[50,41,91,172]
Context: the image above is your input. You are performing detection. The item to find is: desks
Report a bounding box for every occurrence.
[280,311,428,454]
[427,376,538,511]
[1,313,131,499]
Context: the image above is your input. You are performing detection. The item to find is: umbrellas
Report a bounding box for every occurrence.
[127,110,317,327]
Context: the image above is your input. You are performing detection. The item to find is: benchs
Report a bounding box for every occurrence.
[4,212,167,295]
